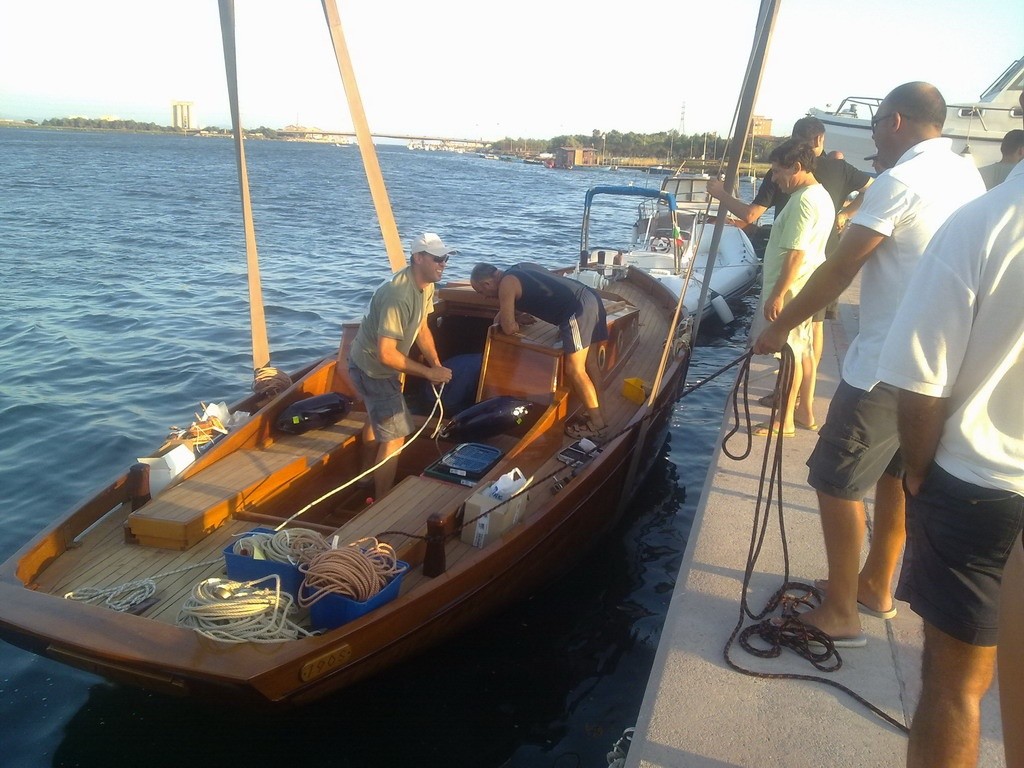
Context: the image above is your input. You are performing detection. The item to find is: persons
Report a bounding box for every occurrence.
[754,80,989,648]
[471,263,612,442]
[347,233,452,501]
[706,121,891,436]
[979,129,1023,190]
[875,89,1024,768]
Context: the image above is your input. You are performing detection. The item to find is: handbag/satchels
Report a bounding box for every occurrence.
[488,467,526,502]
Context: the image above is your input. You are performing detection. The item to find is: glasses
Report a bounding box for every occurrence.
[872,112,911,131]
[426,254,450,263]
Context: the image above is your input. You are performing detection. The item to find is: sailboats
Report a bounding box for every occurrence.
[563,138,612,171]
[480,139,556,168]
[642,151,682,175]
[696,118,758,183]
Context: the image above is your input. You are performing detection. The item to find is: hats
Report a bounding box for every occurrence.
[864,151,880,160]
[411,232,462,257]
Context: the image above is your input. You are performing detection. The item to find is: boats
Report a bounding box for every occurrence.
[639,174,741,219]
[577,184,761,321]
[0,4,700,709]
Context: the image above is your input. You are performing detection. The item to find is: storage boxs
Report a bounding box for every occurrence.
[418,441,506,490]
[136,443,195,497]
[460,475,535,549]
[309,548,409,630]
[222,526,311,601]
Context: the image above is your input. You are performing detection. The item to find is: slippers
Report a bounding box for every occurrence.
[745,423,796,438]
[816,580,898,619]
[773,617,868,647]
[776,406,819,430]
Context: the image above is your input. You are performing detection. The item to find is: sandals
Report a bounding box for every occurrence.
[760,392,800,410]
[564,420,609,442]
[580,410,591,420]
